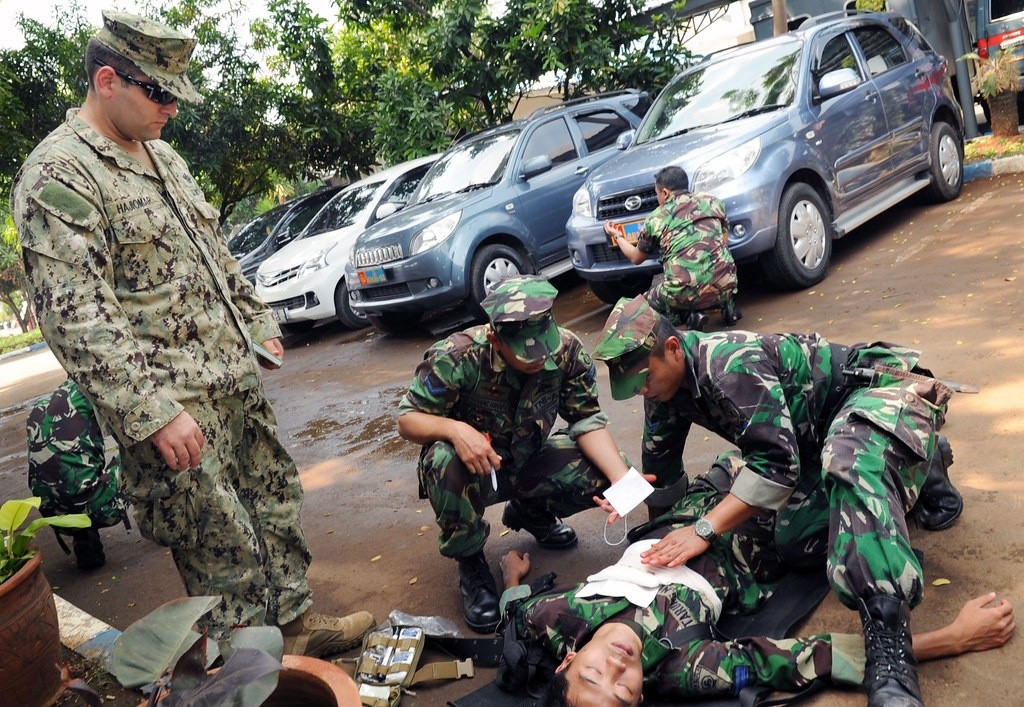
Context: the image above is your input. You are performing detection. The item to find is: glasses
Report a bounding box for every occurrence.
[94,58,177,105]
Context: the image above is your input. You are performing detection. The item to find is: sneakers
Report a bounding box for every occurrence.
[279,607,377,659]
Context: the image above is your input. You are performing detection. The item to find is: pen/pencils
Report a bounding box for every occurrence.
[485,432,498,491]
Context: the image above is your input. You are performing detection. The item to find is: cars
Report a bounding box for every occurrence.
[254,153,448,334]
[226,184,351,288]
[975,0,1024,128]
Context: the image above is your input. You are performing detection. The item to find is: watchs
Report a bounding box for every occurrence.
[695,517,717,544]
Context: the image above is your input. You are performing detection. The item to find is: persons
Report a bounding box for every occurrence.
[26,374,132,572]
[591,295,963,707]
[603,166,744,333]
[495,449,1016,707]
[398,275,656,635]
[9,9,377,660]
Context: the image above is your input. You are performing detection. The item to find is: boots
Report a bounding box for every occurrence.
[686,310,709,332]
[503,505,577,550]
[911,436,963,531]
[454,550,500,635]
[721,298,743,326]
[856,595,924,707]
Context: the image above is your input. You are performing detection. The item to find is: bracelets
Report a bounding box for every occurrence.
[614,232,623,240]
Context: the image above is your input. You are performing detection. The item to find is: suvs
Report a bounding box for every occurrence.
[566,8,967,305]
[344,88,671,340]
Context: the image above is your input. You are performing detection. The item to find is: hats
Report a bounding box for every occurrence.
[594,293,661,400]
[93,9,204,105]
[481,274,564,364]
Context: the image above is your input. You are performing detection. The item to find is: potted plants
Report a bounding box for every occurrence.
[953,46,1021,137]
[110,598,363,707]
[0,495,91,707]
